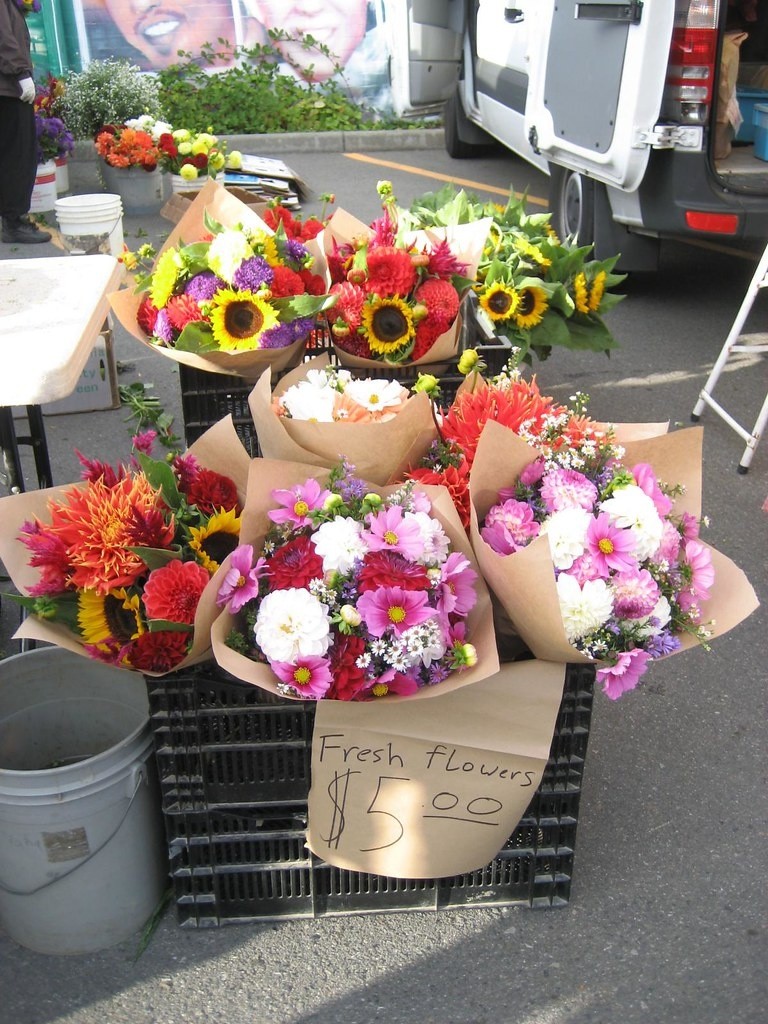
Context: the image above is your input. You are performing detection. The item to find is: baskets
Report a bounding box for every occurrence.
[147,646,598,929]
[182,288,517,459]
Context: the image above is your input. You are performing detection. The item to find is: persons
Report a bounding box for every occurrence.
[251,0,370,81]
[0,0,54,243]
[103,0,234,74]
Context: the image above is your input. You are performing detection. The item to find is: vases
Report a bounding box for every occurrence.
[27,157,58,215]
[101,157,163,218]
[171,173,225,192]
[52,156,69,194]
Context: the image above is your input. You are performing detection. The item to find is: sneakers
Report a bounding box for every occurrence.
[2,219,52,242]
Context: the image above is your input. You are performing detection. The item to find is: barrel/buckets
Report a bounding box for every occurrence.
[27,160,58,213]
[101,158,164,206]
[171,171,225,194]
[55,150,69,195]
[1,646,171,956]
[55,193,124,265]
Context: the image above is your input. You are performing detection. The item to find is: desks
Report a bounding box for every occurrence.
[0,252,127,656]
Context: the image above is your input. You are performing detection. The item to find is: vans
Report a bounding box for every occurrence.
[442,0,768,295]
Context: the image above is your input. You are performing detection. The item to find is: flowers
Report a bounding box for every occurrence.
[15,0,41,17]
[31,55,714,700]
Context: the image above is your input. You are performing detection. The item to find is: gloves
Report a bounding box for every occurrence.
[18,77,36,104]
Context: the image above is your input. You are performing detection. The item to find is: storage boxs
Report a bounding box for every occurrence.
[142,658,599,929]
[751,103,768,161]
[729,81,768,144]
[159,185,271,225]
[179,288,513,459]
[9,311,122,420]
